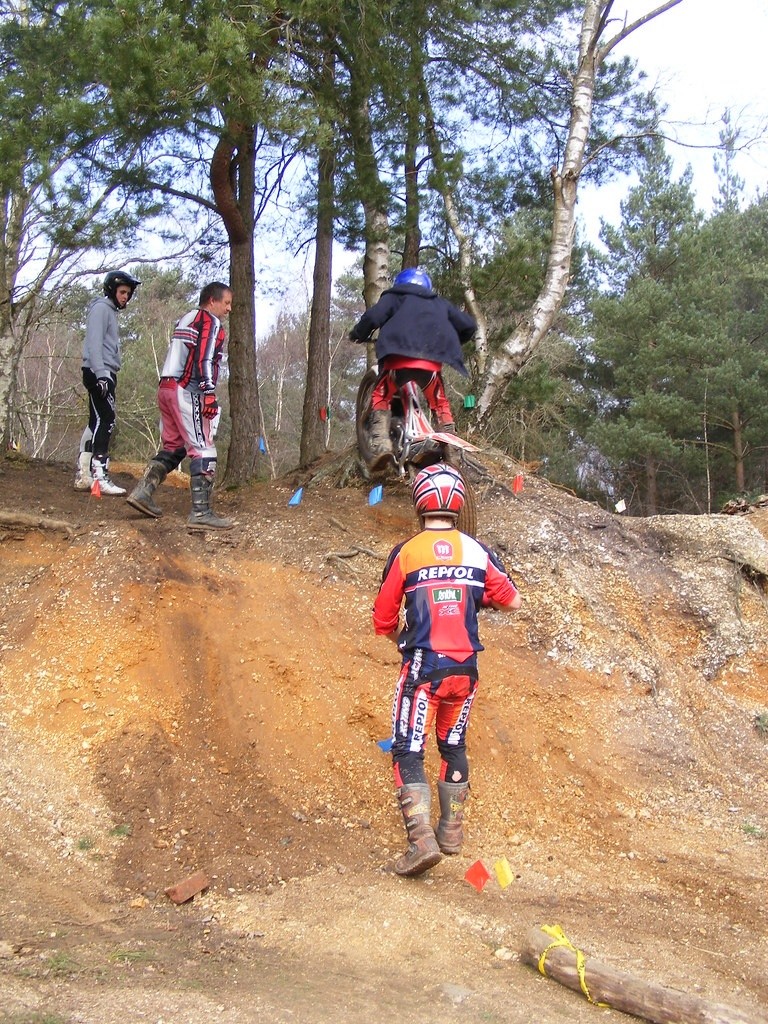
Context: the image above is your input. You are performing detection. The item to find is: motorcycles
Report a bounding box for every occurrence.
[351,336,482,541]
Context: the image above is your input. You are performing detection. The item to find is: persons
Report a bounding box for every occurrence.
[126,282,233,530]
[349,269,477,471]
[74,271,141,495]
[374,463,522,875]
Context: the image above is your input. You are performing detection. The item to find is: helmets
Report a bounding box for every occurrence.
[393,268,432,289]
[103,270,142,310]
[412,464,467,517]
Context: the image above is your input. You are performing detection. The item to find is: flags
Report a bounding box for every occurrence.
[495,858,514,889]
[260,437,265,454]
[614,499,626,513]
[464,861,490,892]
[464,395,476,408]
[321,406,330,421]
[289,489,302,506]
[369,486,382,506]
[92,480,101,498]
[513,474,523,494]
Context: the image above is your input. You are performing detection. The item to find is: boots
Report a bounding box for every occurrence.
[443,422,459,463]
[74,451,127,495]
[368,409,394,474]
[186,458,233,531]
[393,783,442,876]
[127,460,168,516]
[435,777,469,854]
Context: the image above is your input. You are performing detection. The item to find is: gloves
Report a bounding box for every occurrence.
[98,378,116,403]
[201,394,218,420]
[349,330,364,345]
